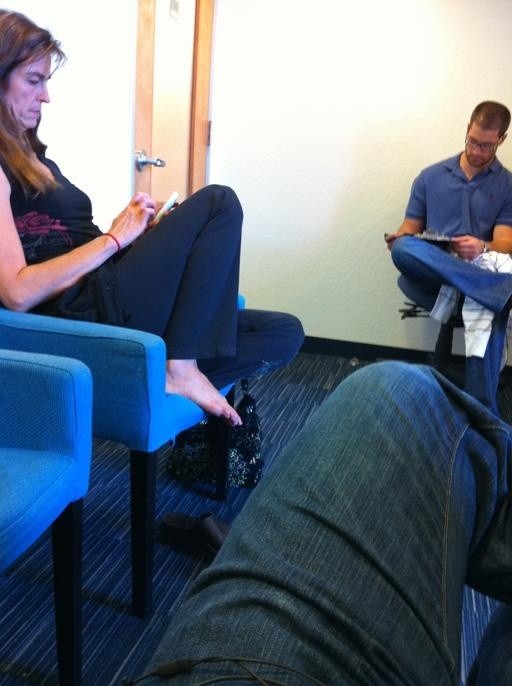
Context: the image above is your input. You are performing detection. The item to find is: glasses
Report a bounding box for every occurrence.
[464,125,504,153]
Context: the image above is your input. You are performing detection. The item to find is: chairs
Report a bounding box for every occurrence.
[0,349,97,686]
[0,306,247,622]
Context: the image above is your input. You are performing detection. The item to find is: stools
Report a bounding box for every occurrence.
[397,273,461,374]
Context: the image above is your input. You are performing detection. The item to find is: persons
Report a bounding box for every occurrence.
[133,360,512,686]
[0,8,245,427]
[382,99,512,417]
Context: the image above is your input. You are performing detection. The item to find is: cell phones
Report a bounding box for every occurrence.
[148,191,180,227]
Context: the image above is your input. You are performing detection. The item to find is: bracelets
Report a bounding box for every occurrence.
[102,232,121,252]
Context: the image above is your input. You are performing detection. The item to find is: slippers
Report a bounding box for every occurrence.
[159,507,234,564]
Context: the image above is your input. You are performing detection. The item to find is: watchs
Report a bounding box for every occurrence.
[482,241,488,253]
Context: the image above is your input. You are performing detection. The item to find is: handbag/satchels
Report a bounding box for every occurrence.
[166,378,265,490]
